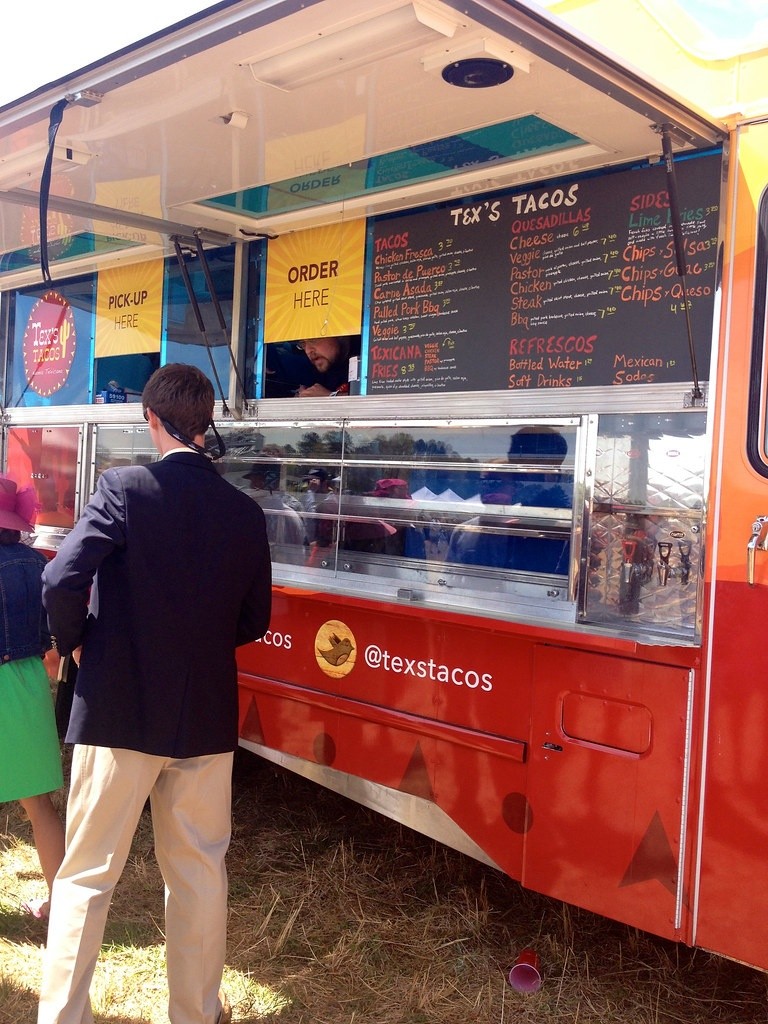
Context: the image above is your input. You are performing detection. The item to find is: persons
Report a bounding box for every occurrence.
[0,472,69,920]
[40,362,274,1023]
[209,426,574,580]
[293,336,352,397]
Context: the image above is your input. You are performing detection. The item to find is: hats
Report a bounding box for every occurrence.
[318,500,398,541]
[1,477,40,533]
[240,464,280,476]
[302,468,326,480]
[373,478,412,500]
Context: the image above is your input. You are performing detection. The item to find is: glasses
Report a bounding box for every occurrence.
[296,339,322,350]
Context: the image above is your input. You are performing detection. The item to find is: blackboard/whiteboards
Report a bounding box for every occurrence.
[361,145,725,398]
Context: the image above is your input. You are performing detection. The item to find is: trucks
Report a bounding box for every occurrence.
[0,0,768,976]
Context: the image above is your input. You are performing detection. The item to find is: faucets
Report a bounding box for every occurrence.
[620,540,638,584]
[656,541,672,587]
[678,540,692,586]
[745,515,768,586]
